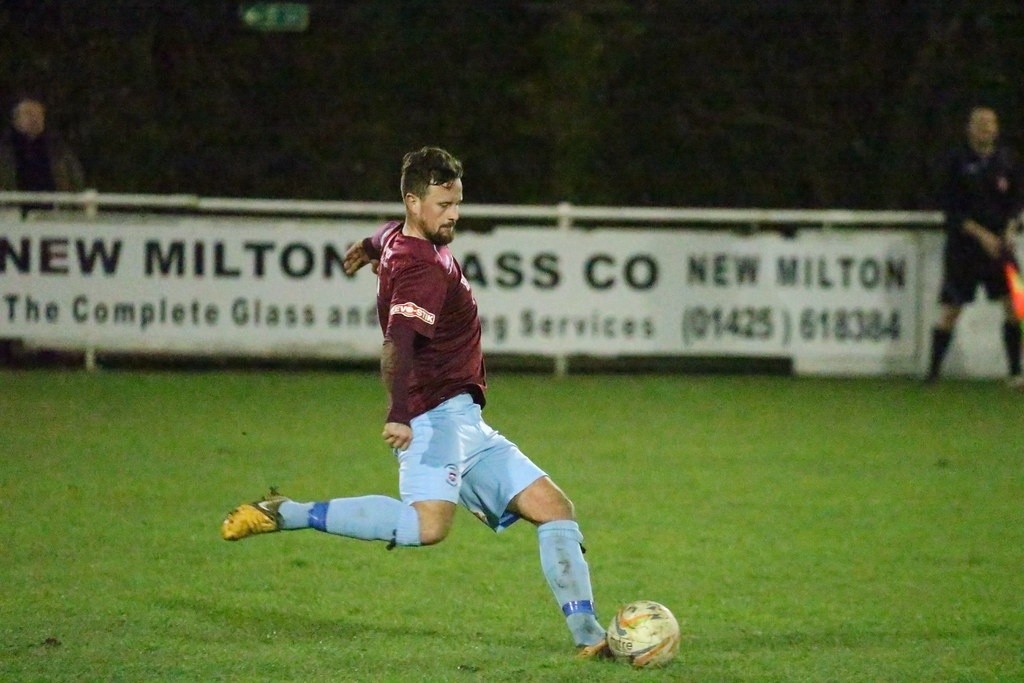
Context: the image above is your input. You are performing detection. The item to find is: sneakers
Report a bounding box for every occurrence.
[222,487,290,540]
[573,640,611,661]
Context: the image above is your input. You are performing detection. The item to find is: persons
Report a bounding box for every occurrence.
[222,148,611,660]
[923,109,1024,391]
[0,98,101,217]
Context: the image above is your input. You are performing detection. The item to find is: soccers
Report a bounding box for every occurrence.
[607,600,680,669]
